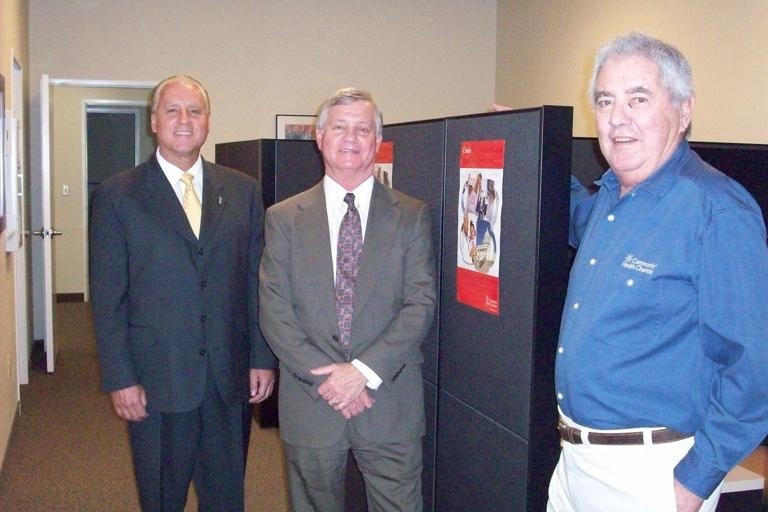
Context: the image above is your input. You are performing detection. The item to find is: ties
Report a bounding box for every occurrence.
[179,172,202,240]
[336,193,362,362]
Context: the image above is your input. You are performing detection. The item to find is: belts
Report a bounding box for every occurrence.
[557,414,695,444]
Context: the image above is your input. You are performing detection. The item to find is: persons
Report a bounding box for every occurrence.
[255,85,441,512]
[491,33,766,511]
[460,171,499,266]
[86,74,280,511]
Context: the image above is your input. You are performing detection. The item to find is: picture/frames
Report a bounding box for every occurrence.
[276,114,318,140]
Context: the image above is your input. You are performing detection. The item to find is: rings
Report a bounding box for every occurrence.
[329,400,338,404]
